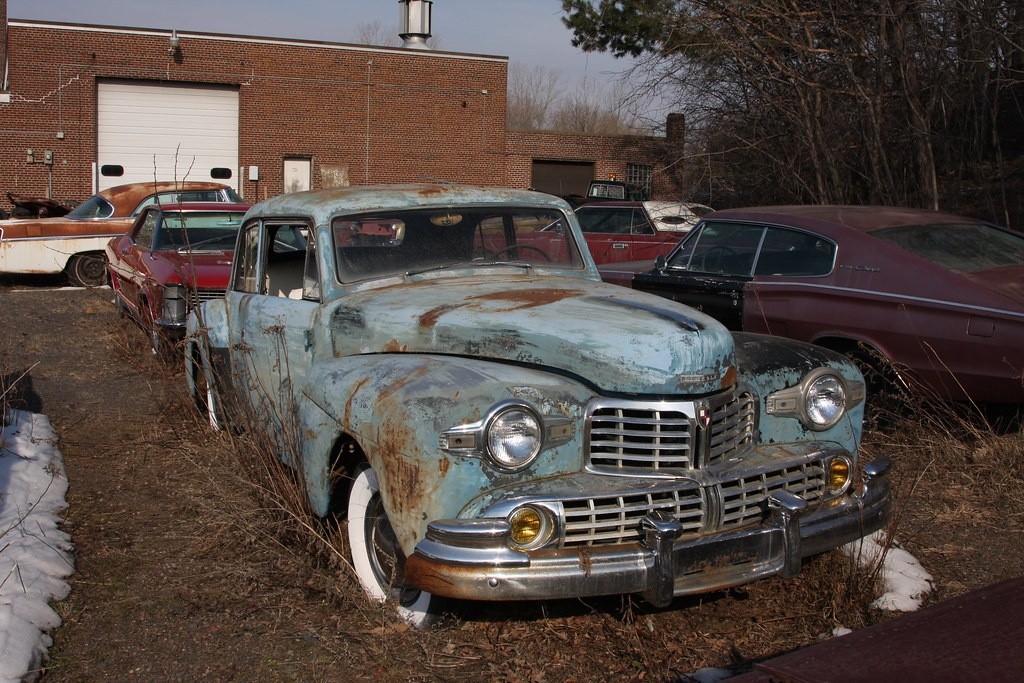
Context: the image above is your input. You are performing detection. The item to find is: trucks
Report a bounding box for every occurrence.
[562,174,649,218]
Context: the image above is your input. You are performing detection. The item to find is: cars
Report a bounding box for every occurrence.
[0,180,252,289]
[104,201,314,370]
[596,203,1024,434]
[183,183,892,628]
[297,215,553,258]
[473,201,791,276]
